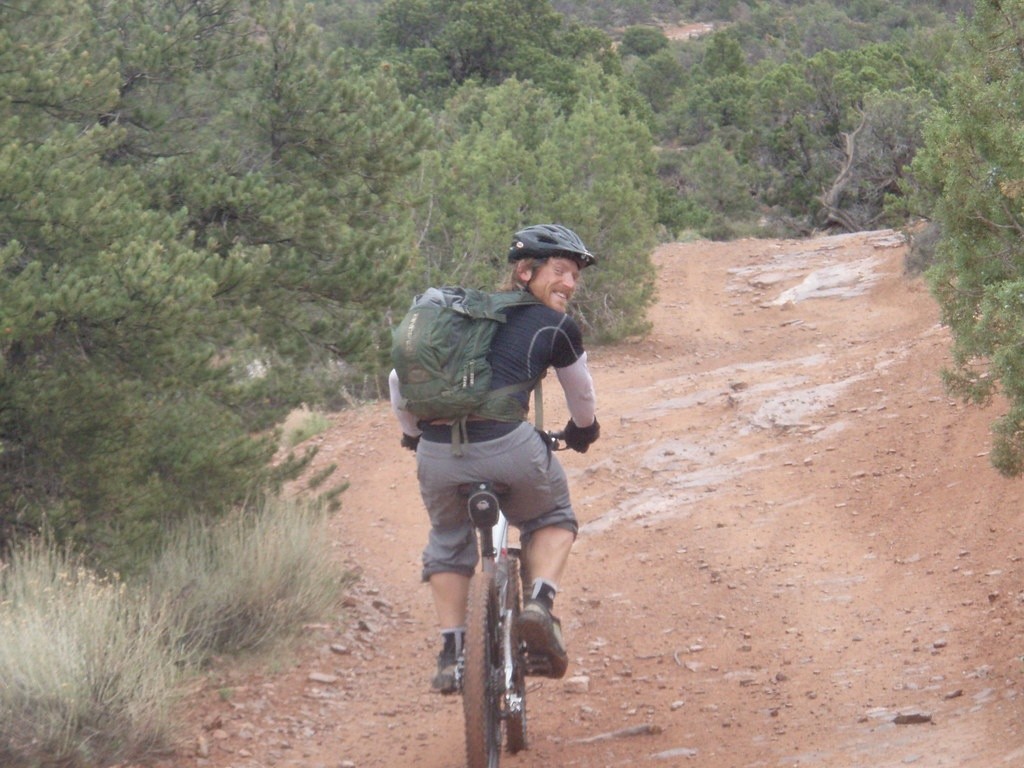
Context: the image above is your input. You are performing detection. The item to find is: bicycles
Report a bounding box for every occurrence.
[401,425,571,768]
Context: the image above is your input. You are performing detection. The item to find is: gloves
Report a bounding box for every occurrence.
[564,414,601,454]
[400,432,423,452]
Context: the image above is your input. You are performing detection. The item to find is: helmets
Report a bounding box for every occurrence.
[507,222,597,271]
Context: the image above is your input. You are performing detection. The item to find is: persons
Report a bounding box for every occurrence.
[388,223,601,692]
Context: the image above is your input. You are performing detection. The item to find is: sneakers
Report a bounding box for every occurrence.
[518,600,569,680]
[427,652,464,698]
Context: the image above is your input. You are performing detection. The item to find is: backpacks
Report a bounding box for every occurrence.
[390,285,546,422]
